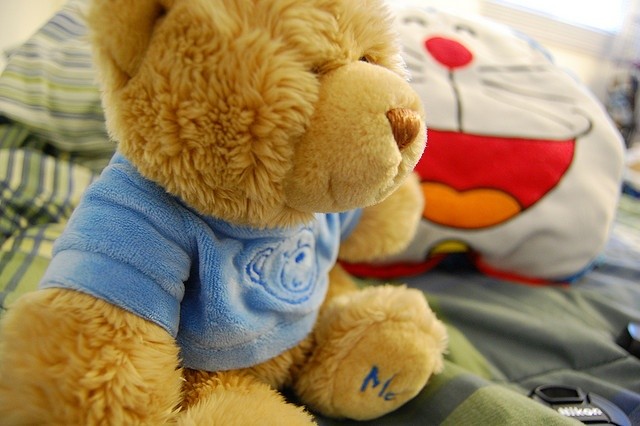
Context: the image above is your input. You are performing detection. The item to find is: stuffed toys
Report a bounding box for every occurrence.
[0,0,444,426]
